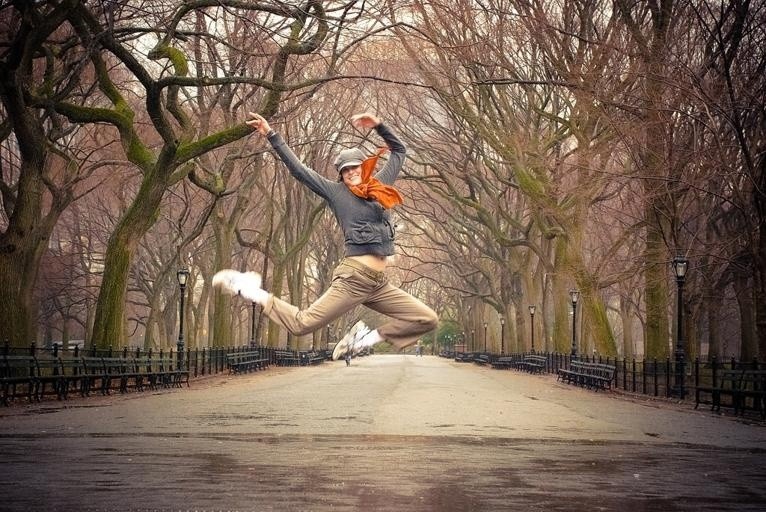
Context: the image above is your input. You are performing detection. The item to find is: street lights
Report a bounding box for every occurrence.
[672,254,690,396]
[528,303,537,354]
[483,321,489,350]
[250,300,257,345]
[444,329,475,354]
[327,323,330,343]
[176,265,189,369]
[500,317,506,356]
[338,327,342,339]
[568,287,580,361]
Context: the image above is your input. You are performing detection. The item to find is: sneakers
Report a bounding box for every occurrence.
[329,320,371,361]
[210,268,263,298]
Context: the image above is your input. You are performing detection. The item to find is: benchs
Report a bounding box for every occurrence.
[557,360,616,392]
[692,368,765,419]
[455,353,547,375]
[0,355,190,408]
[224,350,332,376]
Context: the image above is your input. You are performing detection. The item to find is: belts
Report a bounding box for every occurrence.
[342,257,385,283]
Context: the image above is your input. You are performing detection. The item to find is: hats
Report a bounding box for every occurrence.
[332,147,366,173]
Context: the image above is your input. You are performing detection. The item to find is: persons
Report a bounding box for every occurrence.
[345,355,352,365]
[211,113,439,360]
[414,344,424,357]
